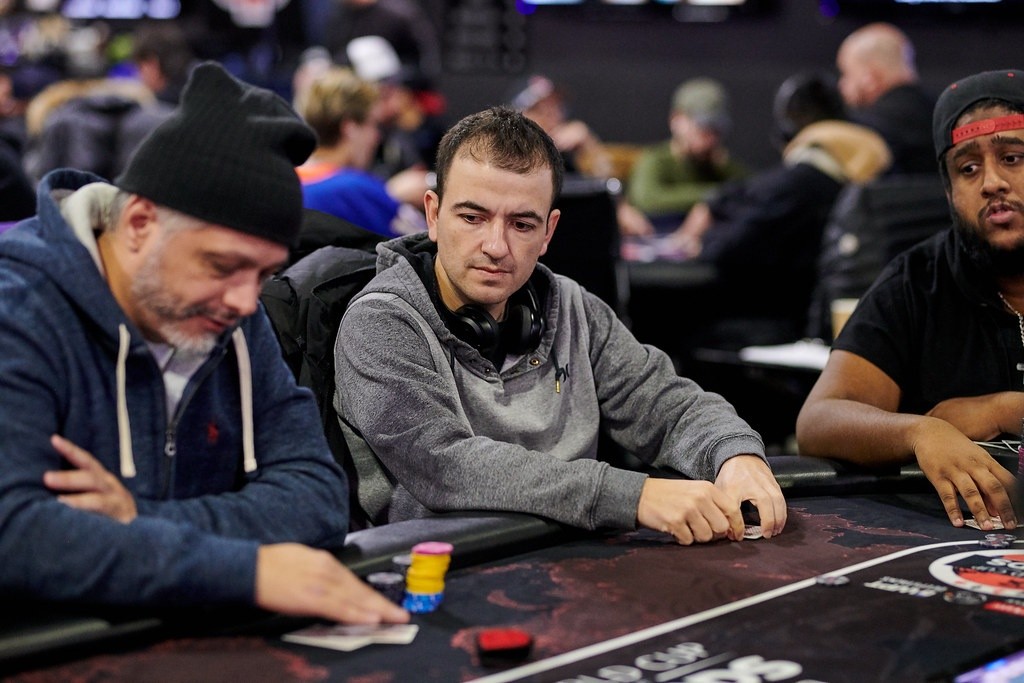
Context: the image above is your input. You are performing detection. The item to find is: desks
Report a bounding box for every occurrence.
[0,452,1024,683]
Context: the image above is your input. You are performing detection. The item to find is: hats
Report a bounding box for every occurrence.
[932,70,1024,162]
[673,76,731,131]
[114,61,319,247]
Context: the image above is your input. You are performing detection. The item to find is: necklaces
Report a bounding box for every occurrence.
[998,290,1024,345]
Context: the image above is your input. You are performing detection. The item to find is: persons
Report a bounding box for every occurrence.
[1,0,1024,629]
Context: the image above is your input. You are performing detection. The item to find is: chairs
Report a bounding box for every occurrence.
[536,175,634,332]
